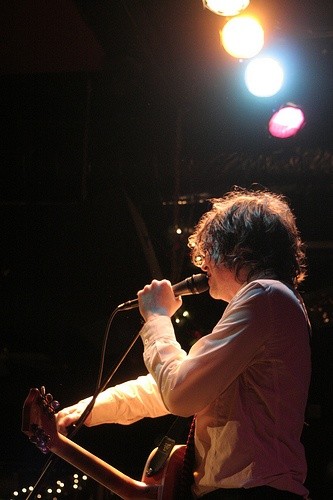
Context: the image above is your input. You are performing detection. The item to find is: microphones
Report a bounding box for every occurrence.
[117,274,209,311]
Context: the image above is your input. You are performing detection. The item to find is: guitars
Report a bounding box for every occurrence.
[20,385,187,500]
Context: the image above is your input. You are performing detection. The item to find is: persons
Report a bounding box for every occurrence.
[54,190,314,500]
